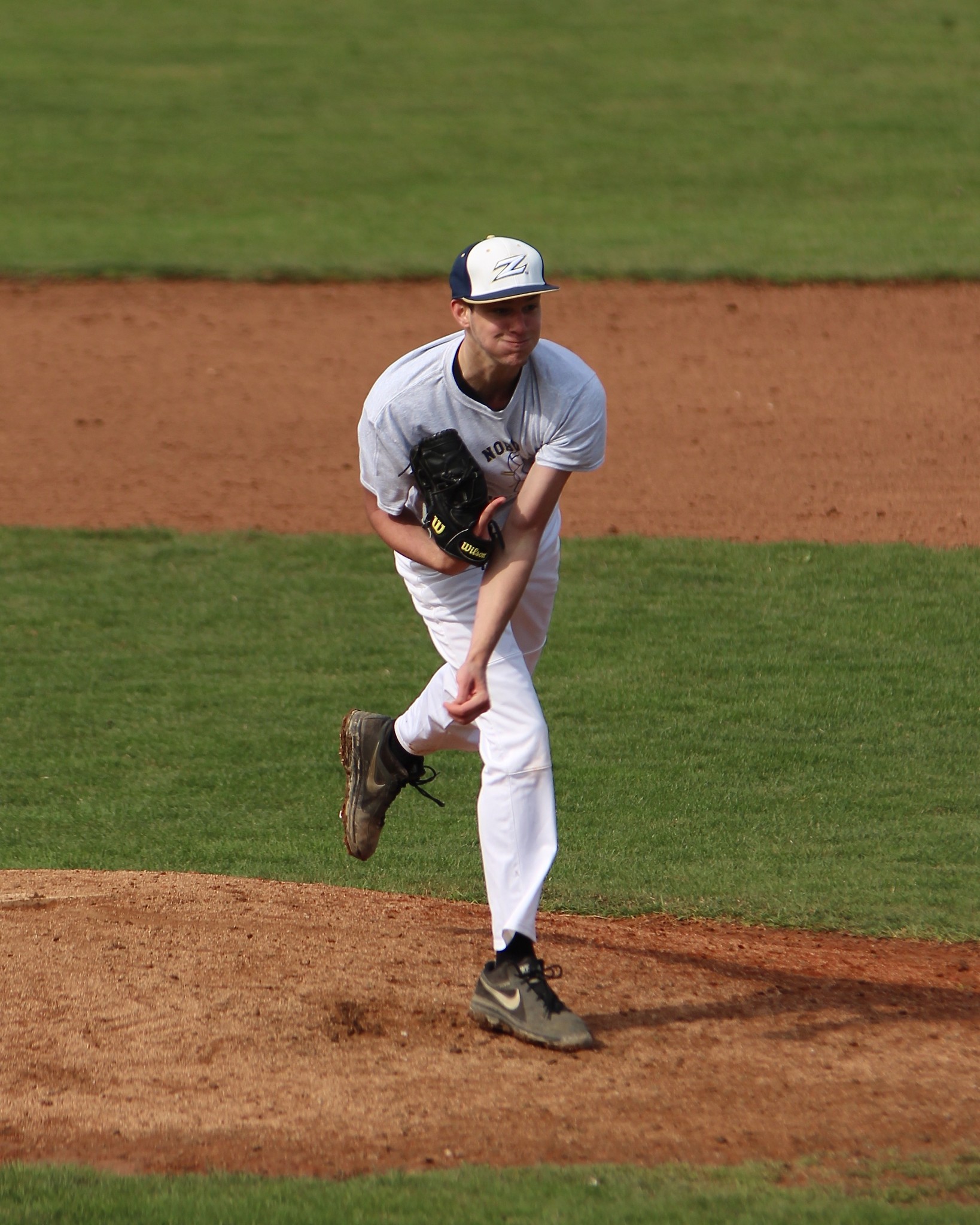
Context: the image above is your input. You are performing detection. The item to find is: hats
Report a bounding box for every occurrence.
[449,235,560,305]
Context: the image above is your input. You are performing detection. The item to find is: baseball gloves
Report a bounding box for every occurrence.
[398,427,506,572]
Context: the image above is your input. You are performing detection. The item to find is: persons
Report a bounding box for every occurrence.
[341,235,606,1050]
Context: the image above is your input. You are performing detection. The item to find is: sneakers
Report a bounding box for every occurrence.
[339,708,444,860]
[466,957,591,1050]
